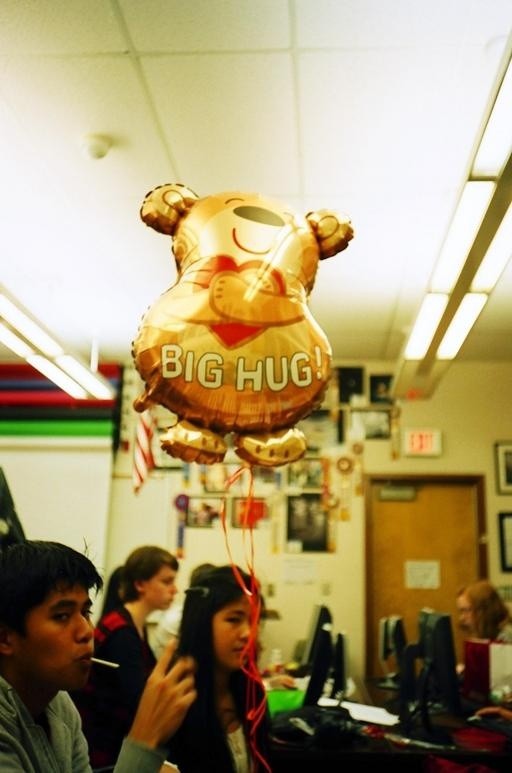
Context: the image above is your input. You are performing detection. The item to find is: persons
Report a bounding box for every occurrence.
[0,541,196,773]
[474,706,511,724]
[161,562,273,773]
[82,546,179,771]
[451,580,511,703]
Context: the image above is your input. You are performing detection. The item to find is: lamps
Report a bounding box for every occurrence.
[0,135,119,401]
[388,27,512,401]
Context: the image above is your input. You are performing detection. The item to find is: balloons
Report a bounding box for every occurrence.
[130,183,357,465]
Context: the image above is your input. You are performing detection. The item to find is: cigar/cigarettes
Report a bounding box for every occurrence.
[89,656,120,671]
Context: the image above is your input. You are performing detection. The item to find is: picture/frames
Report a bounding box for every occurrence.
[499,511,512,572]
[494,438,512,496]
[185,367,398,554]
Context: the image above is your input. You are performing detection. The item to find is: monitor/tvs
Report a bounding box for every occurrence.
[274,603,472,747]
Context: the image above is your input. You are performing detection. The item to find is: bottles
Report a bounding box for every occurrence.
[490,672,512,705]
[270,648,283,674]
